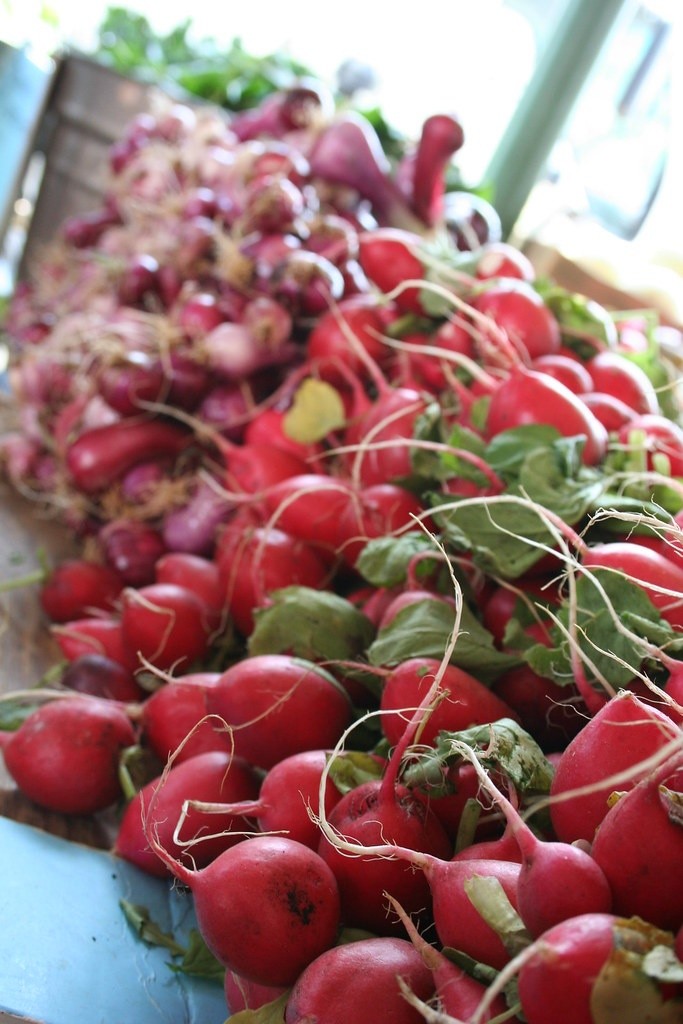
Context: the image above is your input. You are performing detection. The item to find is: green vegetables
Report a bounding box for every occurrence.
[84,4,405,160]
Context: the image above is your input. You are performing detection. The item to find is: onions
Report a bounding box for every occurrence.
[0,88,407,555]
